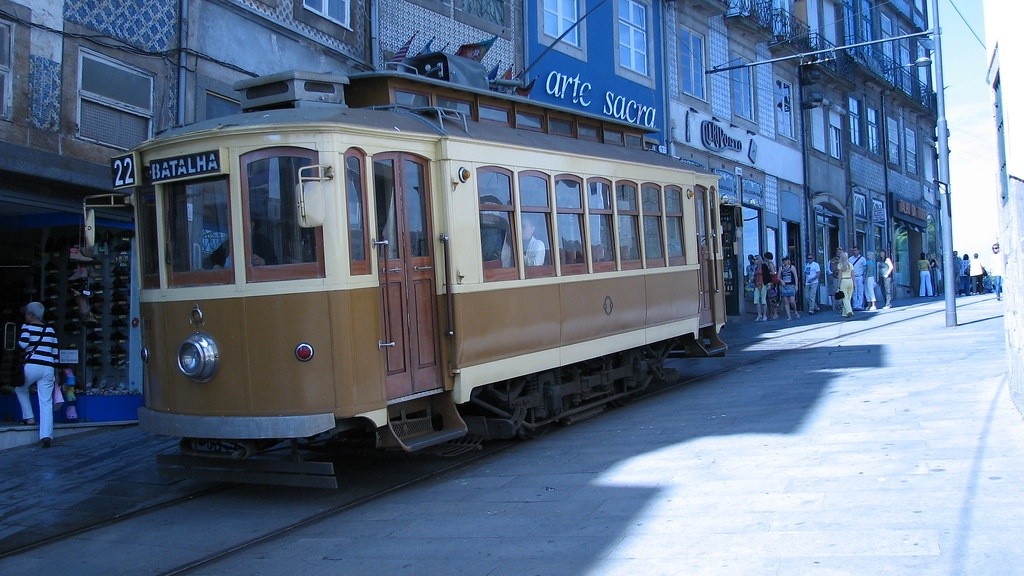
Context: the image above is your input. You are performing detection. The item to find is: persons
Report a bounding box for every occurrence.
[744,244,1002,322]
[14,302,59,447]
[501,216,546,268]
[209,208,279,270]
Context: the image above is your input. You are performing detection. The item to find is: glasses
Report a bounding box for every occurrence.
[992,247,998,250]
[753,258,758,262]
[807,258,812,259]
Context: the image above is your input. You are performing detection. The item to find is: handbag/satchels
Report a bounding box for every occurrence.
[964,269,967,275]
[10,366,25,387]
[835,288,844,300]
[767,262,777,284]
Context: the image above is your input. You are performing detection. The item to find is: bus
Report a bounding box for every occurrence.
[111,50,730,491]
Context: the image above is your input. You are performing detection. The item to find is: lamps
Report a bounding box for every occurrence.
[884,55,932,71]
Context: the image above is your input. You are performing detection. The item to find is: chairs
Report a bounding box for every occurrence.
[483,259,503,270]
[544,245,630,266]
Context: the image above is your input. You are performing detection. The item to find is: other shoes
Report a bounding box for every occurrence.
[64,371,78,421]
[18,418,35,425]
[40,437,52,447]
[762,316,767,322]
[754,315,762,321]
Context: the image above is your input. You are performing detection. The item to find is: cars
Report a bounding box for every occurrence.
[938,269,992,294]
